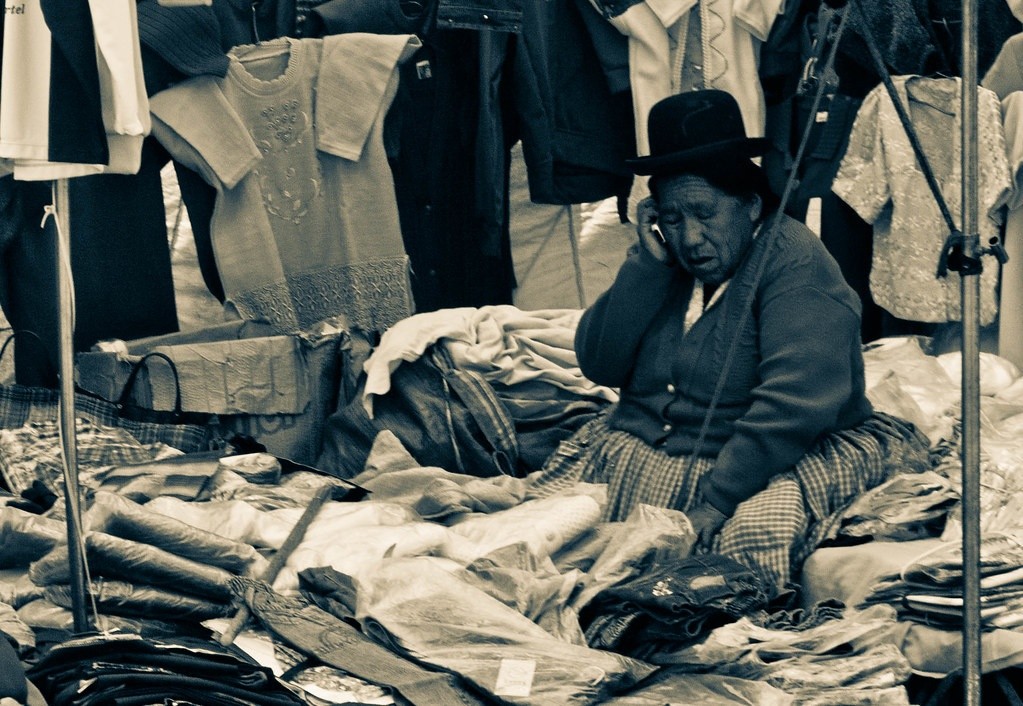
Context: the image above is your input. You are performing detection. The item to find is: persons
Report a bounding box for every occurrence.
[530,90,931,603]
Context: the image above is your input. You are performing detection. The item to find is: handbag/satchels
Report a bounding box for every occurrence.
[0,330,208,453]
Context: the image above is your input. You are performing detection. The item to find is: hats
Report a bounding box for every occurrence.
[625,89,772,176]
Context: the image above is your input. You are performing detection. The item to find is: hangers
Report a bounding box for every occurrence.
[228,2,291,59]
[906,15,960,84]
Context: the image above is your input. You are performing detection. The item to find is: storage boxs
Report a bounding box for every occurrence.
[90,316,310,418]
